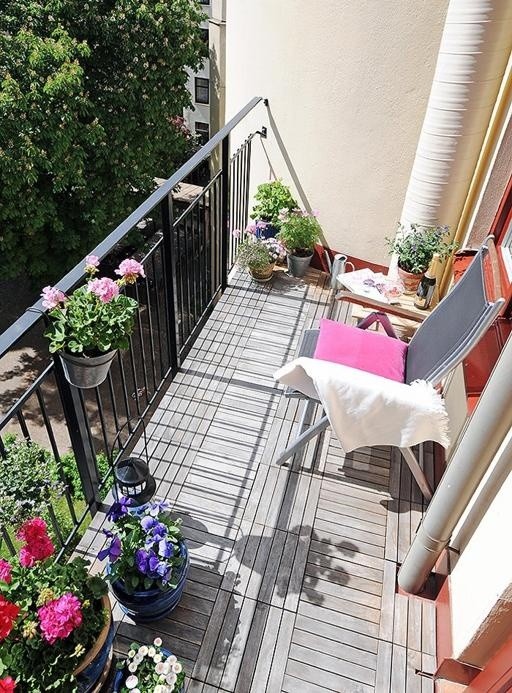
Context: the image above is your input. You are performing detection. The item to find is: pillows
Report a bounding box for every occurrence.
[312,315,410,384]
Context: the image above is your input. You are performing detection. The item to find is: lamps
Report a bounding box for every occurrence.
[109,416,157,515]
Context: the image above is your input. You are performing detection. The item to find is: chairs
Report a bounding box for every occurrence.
[273,232,505,503]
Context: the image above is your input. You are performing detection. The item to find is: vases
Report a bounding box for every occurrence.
[247,261,275,282]
[57,351,117,390]
[395,265,426,295]
[286,252,314,278]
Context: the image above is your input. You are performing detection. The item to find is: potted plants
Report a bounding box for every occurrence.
[249,180,296,239]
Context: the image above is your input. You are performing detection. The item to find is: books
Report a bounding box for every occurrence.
[334,265,400,305]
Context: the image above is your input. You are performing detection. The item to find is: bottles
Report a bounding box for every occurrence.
[413,253,441,311]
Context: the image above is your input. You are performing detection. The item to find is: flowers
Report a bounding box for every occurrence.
[37,251,149,362]
[385,221,466,273]
[231,206,320,270]
[0,498,190,693]
